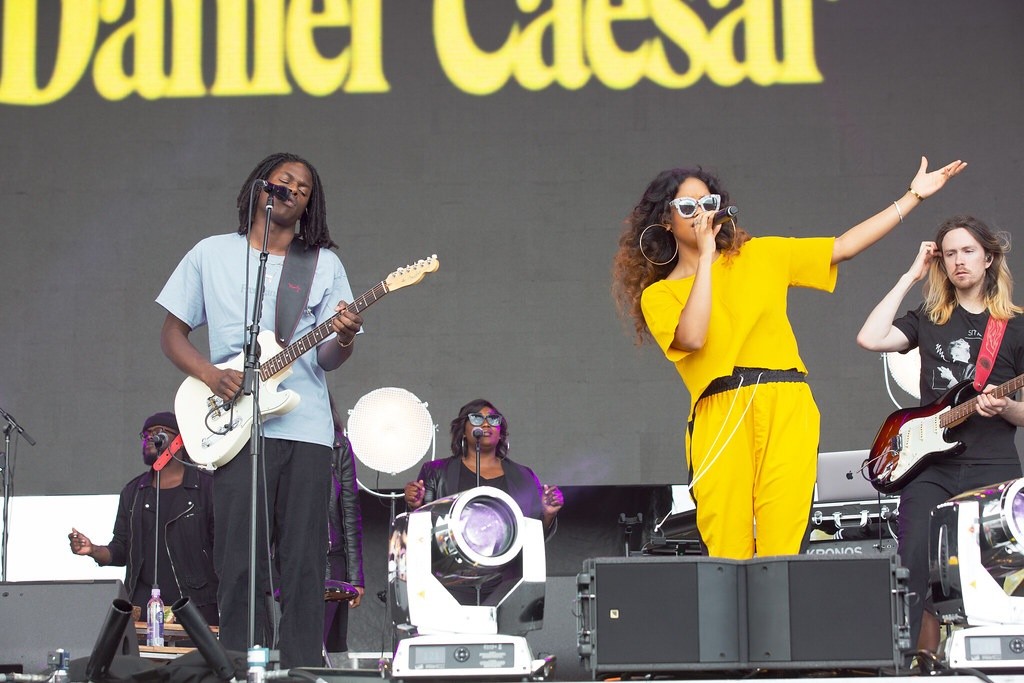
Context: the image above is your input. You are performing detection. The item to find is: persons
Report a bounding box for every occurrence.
[154,152,364,670]
[405,399,564,537]
[68,411,219,647]
[611,157,968,560]
[856,217,1024,670]
[267,389,364,653]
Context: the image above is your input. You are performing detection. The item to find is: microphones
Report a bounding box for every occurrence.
[471,427,483,439]
[153,432,169,443]
[712,206,738,227]
[255,179,291,201]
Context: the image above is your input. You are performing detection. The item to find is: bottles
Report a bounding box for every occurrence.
[146,589,165,647]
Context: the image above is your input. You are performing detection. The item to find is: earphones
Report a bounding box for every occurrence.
[986,255,992,263]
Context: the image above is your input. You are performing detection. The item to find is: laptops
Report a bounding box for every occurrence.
[817,449,884,502]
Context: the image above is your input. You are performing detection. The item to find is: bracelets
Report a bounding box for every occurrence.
[894,201,903,224]
[336,334,356,347]
[908,188,923,201]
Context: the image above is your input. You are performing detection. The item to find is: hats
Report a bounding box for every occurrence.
[143,412,179,431]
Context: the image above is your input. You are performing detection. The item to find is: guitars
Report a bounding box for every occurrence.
[173,251,442,471]
[867,374,1024,495]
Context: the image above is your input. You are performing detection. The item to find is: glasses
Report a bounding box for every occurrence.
[467,412,502,427]
[669,194,721,219]
[139,427,178,440]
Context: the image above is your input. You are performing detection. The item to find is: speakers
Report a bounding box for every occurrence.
[0,579,140,676]
[579,551,911,672]
